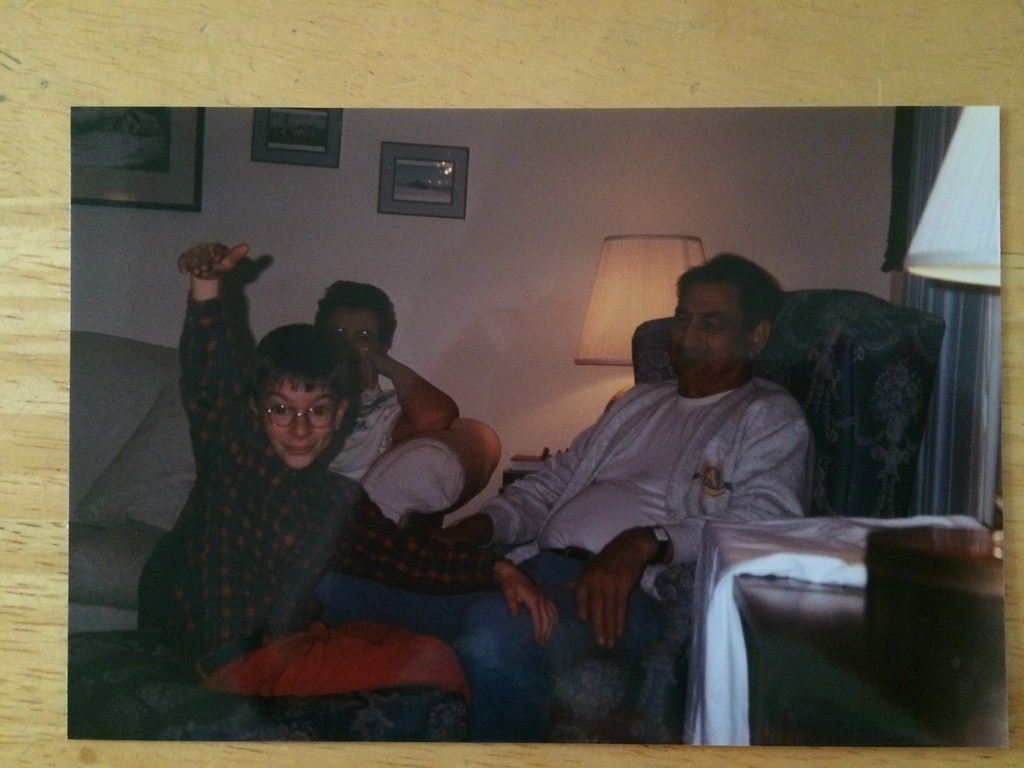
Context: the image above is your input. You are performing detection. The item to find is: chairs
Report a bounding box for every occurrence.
[550,287,946,746]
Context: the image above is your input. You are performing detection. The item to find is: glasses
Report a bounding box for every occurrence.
[328,327,379,344]
[260,403,339,429]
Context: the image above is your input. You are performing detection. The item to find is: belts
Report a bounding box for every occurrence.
[560,545,594,561]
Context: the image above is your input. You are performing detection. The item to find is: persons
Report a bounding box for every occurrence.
[70,238,560,743]
[426,251,815,744]
[312,281,460,482]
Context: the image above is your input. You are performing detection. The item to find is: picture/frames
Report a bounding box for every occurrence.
[376,140,471,221]
[70,105,206,214]
[251,106,344,169]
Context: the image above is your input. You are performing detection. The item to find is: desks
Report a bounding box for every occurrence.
[679,513,991,746]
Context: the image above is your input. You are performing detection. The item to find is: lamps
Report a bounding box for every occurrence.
[907,103,1000,534]
[575,232,710,369]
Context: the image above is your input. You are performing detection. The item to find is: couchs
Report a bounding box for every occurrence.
[70,328,243,637]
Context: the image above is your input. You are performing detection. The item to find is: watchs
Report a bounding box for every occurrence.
[646,523,673,561]
